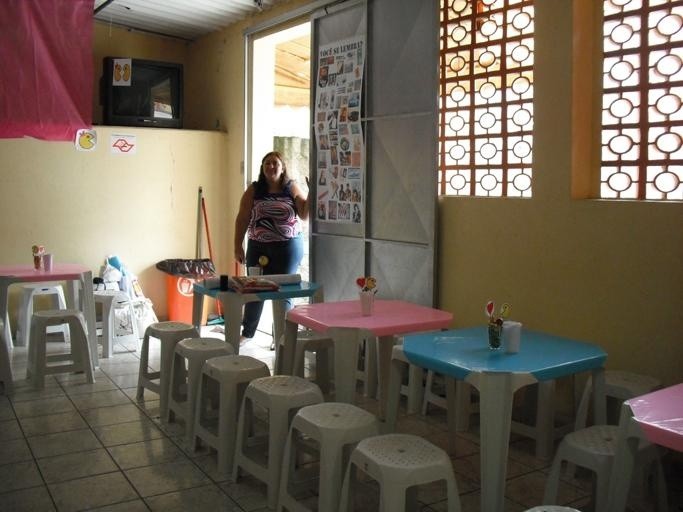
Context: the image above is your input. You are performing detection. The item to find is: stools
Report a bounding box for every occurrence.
[0,321,16,394]
[92,290,140,358]
[195,352,271,482]
[571,365,663,432]
[232,374,324,511]
[26,310,95,392]
[276,401,378,510]
[141,322,193,419]
[171,334,231,430]
[543,424,664,511]
[339,433,462,511]
[19,285,71,347]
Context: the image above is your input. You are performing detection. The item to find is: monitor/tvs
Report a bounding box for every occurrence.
[99,57,184,130]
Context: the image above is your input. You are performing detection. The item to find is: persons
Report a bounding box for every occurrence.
[234,151,310,350]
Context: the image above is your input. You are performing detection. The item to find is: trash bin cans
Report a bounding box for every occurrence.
[163,257,212,327]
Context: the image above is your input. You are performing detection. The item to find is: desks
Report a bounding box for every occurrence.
[386,325,606,510]
[0,265,98,369]
[192,279,324,356]
[609,381,683,512]
[283,300,453,421]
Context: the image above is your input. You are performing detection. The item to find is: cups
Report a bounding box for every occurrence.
[487,317,504,350]
[358,291,373,317]
[247,267,260,277]
[219,274,228,291]
[42,253,53,273]
